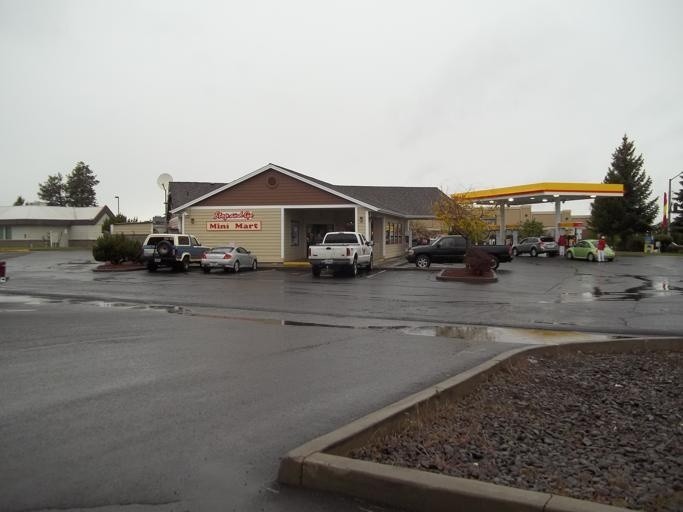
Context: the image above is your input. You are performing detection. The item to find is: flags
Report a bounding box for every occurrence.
[661,193,667,228]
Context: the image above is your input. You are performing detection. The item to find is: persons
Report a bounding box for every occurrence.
[597,235,607,262]
[557,235,576,257]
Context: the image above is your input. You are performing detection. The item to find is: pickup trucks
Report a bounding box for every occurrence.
[404,234,512,271]
[305,230,376,277]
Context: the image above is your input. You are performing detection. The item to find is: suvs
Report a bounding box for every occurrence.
[139,232,213,273]
[509,236,560,258]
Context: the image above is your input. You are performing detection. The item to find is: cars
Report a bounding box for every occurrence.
[665,240,682,252]
[565,238,616,261]
[200,245,258,272]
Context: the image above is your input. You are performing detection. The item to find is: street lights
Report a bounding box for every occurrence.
[667,170,682,244]
[112,195,120,218]
